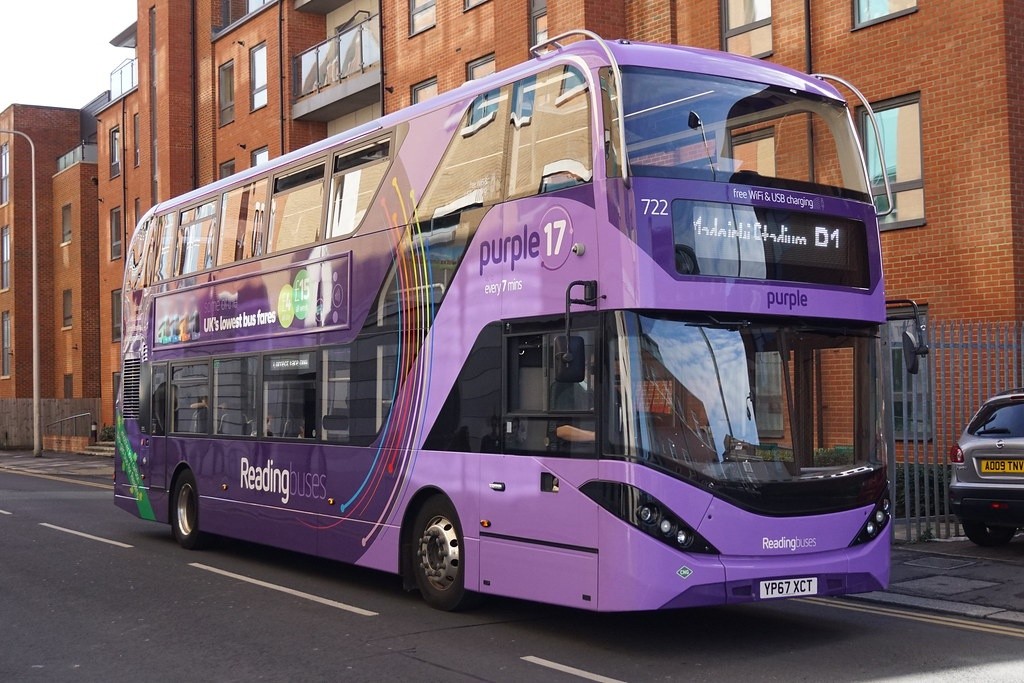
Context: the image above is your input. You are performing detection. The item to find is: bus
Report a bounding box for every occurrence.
[111,29,930,614]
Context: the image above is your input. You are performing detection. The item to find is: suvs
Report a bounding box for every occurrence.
[949,387,1024,547]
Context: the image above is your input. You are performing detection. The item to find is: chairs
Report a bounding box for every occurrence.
[194,409,207,433]
[218,412,248,434]
[547,381,592,449]
[283,419,305,438]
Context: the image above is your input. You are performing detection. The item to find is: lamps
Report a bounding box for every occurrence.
[91,421,97,431]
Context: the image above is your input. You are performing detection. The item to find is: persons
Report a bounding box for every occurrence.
[480,414,523,454]
[190,386,226,418]
[548,349,595,443]
